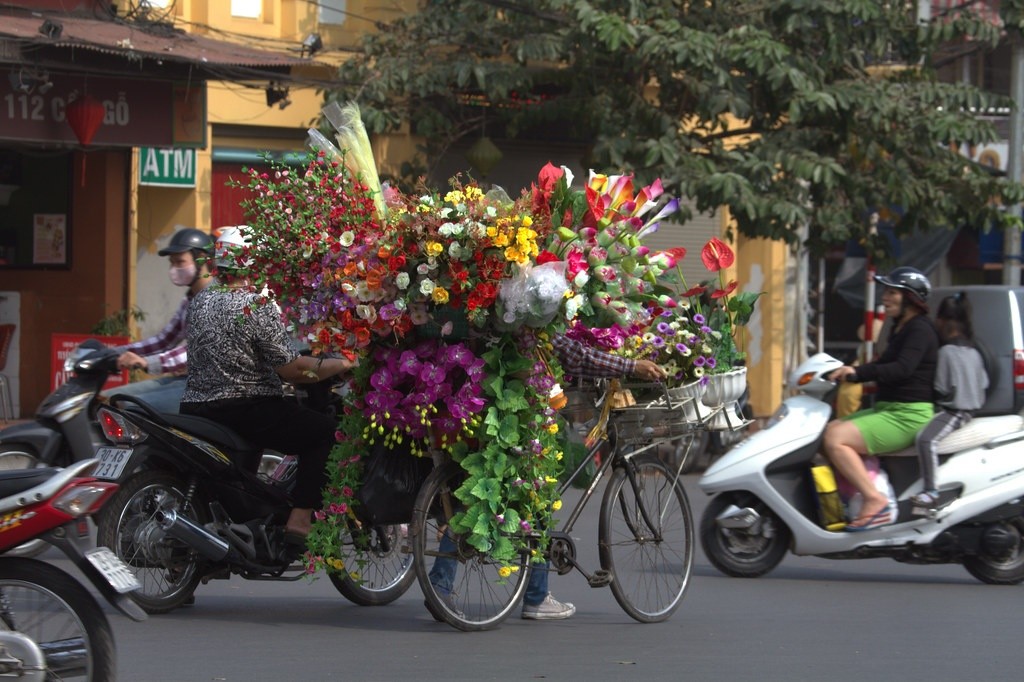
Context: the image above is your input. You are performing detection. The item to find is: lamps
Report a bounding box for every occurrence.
[304,31,323,58]
[9,66,53,95]
[37,18,64,39]
[265,80,290,109]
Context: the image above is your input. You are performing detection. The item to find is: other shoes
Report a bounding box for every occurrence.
[910,491,942,509]
[283,530,341,559]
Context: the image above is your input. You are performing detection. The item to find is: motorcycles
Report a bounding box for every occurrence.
[0,339,284,682]
[91,347,430,616]
[696,348,1024,588]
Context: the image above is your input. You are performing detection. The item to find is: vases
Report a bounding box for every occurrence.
[661,376,713,424]
[702,365,748,429]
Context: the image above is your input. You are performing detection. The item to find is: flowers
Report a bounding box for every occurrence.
[193,138,768,587]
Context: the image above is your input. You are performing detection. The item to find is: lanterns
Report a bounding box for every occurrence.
[66,94,106,185]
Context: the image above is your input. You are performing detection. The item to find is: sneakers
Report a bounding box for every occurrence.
[521,589,576,620]
[421,594,466,620]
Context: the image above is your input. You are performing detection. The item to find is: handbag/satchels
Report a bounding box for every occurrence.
[810,458,900,530]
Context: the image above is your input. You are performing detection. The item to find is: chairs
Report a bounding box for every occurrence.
[0,323,17,422]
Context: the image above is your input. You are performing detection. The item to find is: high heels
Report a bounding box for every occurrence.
[844,505,891,531]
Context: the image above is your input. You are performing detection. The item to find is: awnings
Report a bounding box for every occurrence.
[0,6,310,66]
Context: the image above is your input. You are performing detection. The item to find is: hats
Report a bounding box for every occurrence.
[938,291,968,321]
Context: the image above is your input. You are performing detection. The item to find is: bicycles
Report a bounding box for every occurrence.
[399,374,757,634]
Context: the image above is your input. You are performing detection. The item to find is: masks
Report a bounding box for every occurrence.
[168,265,196,285]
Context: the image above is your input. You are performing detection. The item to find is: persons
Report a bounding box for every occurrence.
[908,291,989,508]
[100,229,218,413]
[424,335,665,620]
[822,267,938,531]
[180,225,359,545]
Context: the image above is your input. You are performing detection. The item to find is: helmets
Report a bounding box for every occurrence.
[214,224,262,269]
[158,229,213,257]
[872,266,931,302]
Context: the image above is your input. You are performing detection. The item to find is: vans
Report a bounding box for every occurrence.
[926,284,1024,419]
